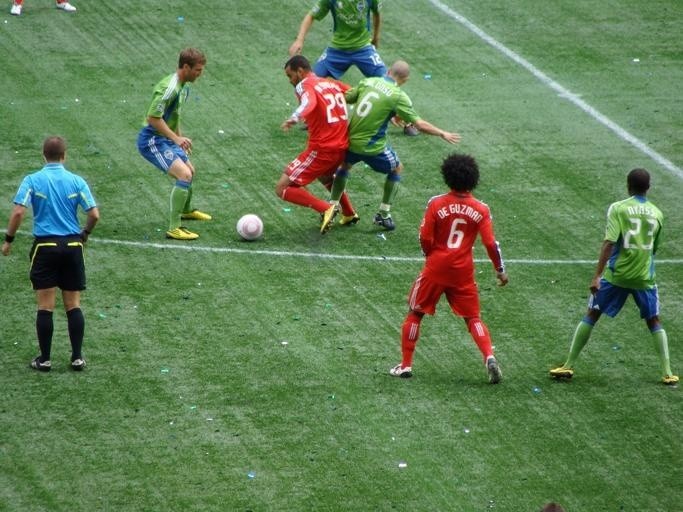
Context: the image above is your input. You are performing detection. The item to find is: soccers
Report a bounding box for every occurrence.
[236,214,263,241]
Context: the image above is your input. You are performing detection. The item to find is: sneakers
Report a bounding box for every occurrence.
[550,367,573,377]
[71,358,86,370]
[181,209,212,220]
[56,1,76,11]
[29,356,51,372]
[662,376,679,384]
[373,213,395,230]
[11,2,22,15]
[486,356,502,384]
[403,123,418,135]
[339,214,360,225]
[320,205,339,235]
[166,227,199,240]
[390,363,412,377]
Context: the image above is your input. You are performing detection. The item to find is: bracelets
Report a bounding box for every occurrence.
[5,234,14,244]
[84,228,92,235]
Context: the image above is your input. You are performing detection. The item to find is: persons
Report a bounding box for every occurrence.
[324,60,463,229]
[10,0,77,15]
[1,136,99,372]
[549,168,680,385]
[288,0,420,135]
[389,152,509,385]
[138,48,213,239]
[274,55,361,234]
